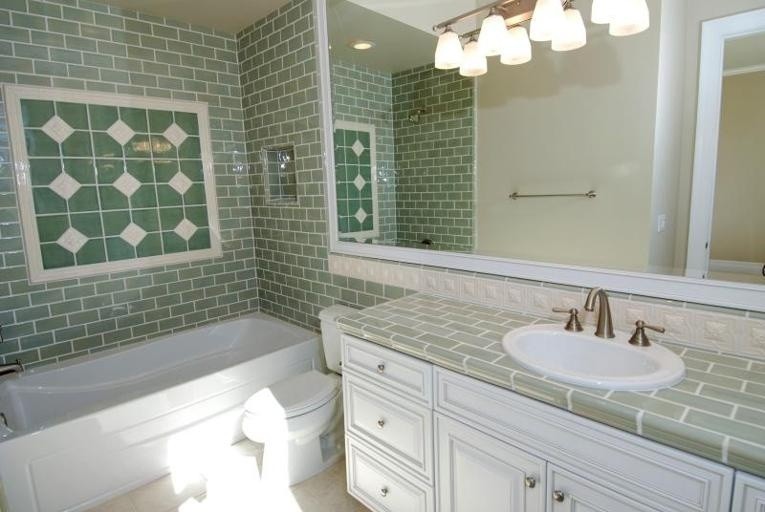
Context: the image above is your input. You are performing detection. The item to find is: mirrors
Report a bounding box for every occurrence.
[311,1,765,314]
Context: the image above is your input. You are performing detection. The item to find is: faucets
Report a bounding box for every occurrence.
[584,286,616,340]
[0,361,26,378]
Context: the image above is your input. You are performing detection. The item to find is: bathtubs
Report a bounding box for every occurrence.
[0,311,329,511]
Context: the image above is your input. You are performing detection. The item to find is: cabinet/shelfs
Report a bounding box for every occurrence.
[341,331,764,512]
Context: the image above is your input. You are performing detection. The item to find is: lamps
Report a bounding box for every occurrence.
[431,1,650,79]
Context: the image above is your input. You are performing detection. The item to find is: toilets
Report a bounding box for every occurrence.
[239,305,364,487]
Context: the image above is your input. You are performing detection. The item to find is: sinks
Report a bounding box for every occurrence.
[502,322,687,393]
